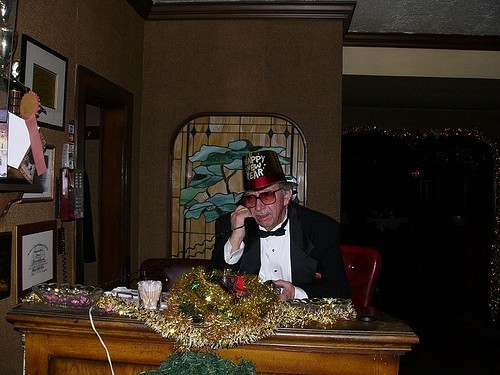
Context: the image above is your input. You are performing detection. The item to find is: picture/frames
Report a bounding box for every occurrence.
[15,219,58,305]
[20,33,68,133]
[17,144,56,204]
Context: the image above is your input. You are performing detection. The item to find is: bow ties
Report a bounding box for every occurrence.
[258,214,289,238]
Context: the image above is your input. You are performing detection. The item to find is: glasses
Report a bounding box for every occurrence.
[243,187,283,209]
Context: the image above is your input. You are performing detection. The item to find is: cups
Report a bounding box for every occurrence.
[137,280,162,311]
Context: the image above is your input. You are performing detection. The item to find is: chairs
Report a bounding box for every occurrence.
[338,244,382,308]
[139,258,210,291]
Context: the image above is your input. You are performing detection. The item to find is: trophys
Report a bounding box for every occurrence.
[0,0,19,80]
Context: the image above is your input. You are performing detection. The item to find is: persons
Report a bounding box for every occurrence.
[209,149,352,302]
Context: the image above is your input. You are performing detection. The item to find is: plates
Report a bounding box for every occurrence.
[32,283,103,308]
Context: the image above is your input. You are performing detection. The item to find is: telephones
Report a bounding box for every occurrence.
[235,204,259,238]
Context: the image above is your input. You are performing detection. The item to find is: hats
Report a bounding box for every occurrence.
[234,150,299,197]
